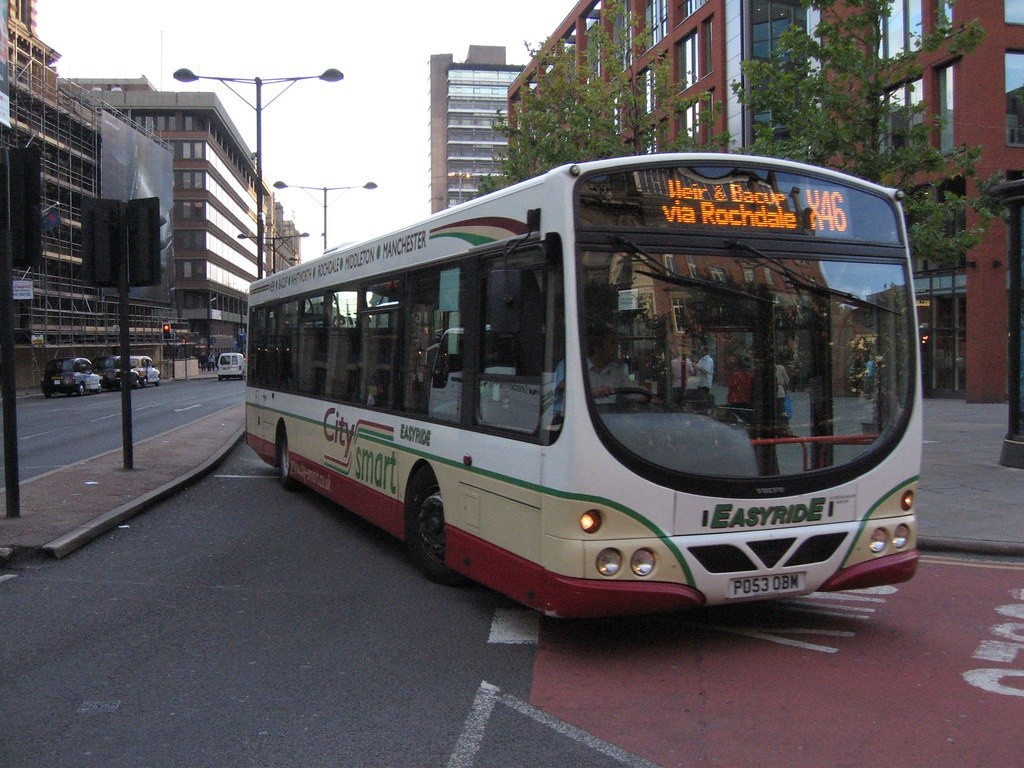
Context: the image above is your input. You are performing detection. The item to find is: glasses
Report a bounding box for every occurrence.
[601,343,620,353]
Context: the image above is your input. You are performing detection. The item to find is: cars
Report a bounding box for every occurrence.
[130,356,162,387]
[95,356,139,390]
[256,334,289,372]
[40,357,101,396]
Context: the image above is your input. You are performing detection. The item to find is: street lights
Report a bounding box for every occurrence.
[447,172,473,205]
[175,67,343,279]
[236,234,311,274]
[272,181,377,254]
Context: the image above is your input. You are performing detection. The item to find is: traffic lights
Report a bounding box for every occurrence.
[163,324,171,339]
[131,197,169,289]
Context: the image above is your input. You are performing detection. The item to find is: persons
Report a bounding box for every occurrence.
[138,367,149,387]
[672,343,713,399]
[727,356,752,420]
[862,354,875,399]
[549,329,662,446]
[197,351,230,372]
[775,354,802,422]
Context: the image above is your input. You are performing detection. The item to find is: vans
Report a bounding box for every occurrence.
[218,353,245,381]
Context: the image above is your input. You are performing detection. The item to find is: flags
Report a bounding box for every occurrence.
[41,208,62,230]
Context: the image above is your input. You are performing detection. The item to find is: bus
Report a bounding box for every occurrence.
[419,329,563,434]
[242,151,929,626]
